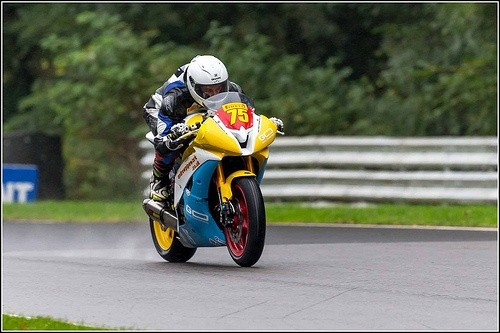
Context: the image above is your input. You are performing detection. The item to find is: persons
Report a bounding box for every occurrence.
[142,55,284,202]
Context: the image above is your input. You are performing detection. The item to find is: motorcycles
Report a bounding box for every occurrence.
[141,92,284,267]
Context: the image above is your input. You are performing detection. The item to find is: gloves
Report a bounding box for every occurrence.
[269,117,284,135]
[170,123,191,139]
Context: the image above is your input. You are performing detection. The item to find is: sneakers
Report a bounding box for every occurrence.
[149,175,173,201]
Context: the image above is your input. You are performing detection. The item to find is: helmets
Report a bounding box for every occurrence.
[186,55,229,110]
